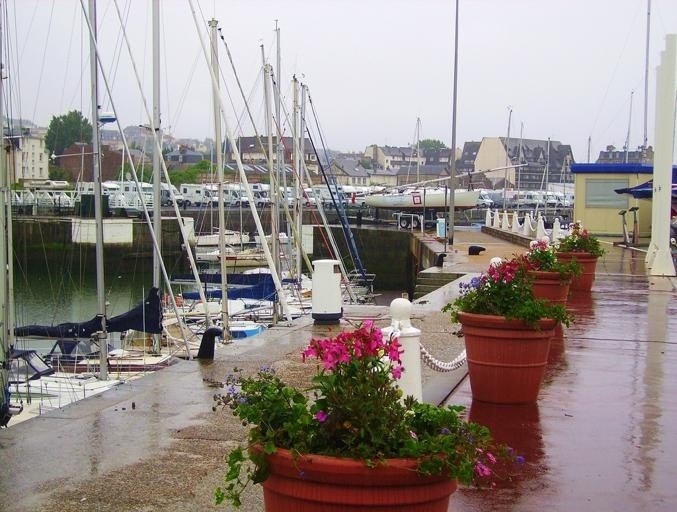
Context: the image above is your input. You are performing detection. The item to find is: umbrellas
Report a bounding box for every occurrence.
[614,169,677,200]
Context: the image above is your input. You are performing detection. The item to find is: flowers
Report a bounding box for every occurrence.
[444,226,613,341]
[211,320,525,506]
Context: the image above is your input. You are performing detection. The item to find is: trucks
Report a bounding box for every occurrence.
[477,191,574,208]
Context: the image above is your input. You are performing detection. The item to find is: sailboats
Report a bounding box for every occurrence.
[365,117,480,207]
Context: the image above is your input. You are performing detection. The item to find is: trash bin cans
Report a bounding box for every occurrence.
[310,258,345,326]
[435,218,446,239]
